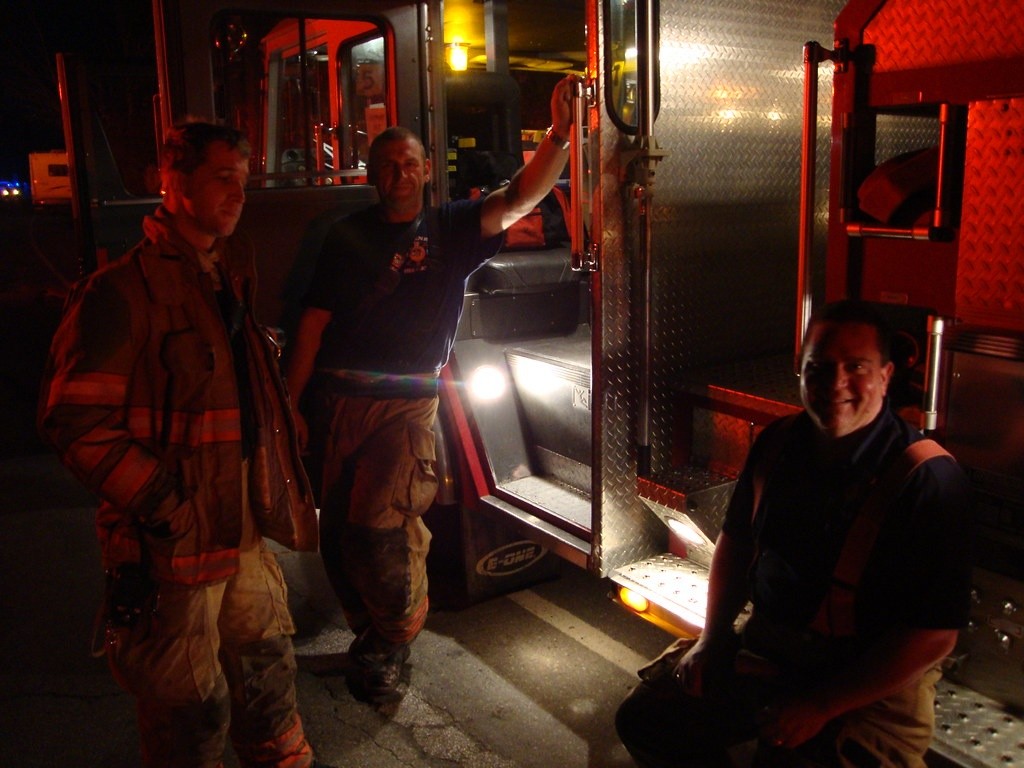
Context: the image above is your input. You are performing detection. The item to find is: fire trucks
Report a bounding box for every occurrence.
[56,0,1024,768]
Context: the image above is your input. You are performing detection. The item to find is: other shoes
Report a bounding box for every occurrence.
[346,626,402,698]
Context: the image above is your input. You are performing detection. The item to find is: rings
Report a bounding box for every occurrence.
[677,672,680,678]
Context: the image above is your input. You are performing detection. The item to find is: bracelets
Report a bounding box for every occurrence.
[546,125,572,151]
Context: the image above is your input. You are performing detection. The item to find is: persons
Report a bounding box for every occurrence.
[39,120,338,768]
[275,73,585,706]
[614,299,985,768]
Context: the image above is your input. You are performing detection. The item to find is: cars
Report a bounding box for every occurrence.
[0,182,23,198]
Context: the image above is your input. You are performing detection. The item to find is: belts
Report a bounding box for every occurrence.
[317,372,439,400]
